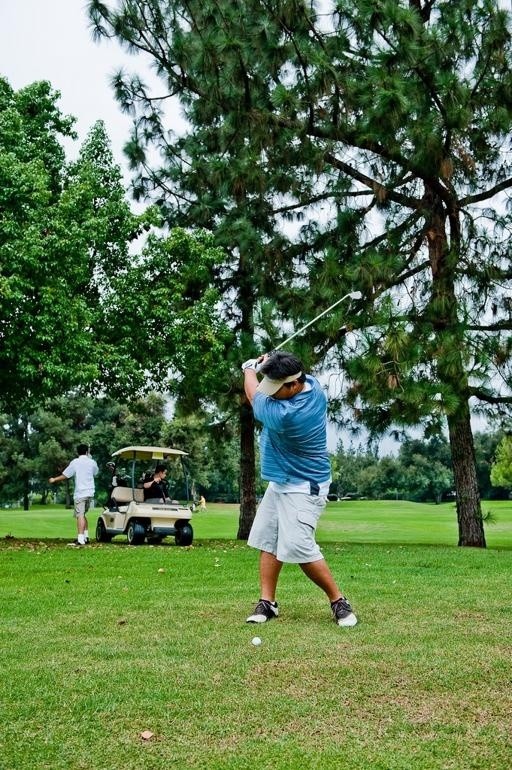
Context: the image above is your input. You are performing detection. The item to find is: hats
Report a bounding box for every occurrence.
[255,370,303,396]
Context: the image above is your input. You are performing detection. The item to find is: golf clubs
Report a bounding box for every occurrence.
[255,290,363,365]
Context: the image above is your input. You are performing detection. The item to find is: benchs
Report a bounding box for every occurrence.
[110,486,146,512]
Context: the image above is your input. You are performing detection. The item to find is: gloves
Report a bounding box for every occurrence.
[241,357,262,374]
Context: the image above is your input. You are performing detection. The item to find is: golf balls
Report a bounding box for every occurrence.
[251,636,262,646]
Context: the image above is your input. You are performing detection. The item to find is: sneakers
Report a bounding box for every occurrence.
[246,598,279,623]
[329,595,358,628]
[72,536,91,546]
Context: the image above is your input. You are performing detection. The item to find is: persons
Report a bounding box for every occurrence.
[49,444,100,546]
[144,465,170,500]
[112,467,127,487]
[242,353,358,627]
[120,468,134,482]
[198,496,208,512]
